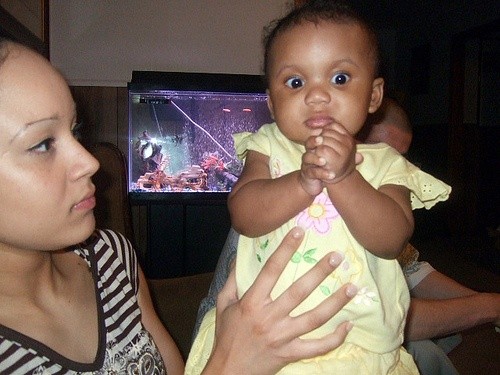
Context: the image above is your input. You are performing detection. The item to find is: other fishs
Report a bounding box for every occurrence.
[243,108,251,112]
[223,108,231,112]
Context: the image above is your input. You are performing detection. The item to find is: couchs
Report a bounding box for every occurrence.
[147,244,500,375]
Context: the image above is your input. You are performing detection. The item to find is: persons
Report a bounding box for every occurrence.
[183,3,456,375]
[0,35,358,375]
[192,95,500,375]
